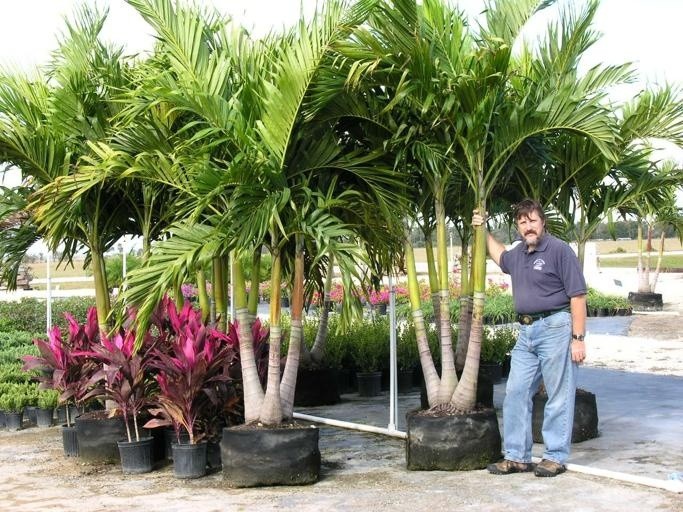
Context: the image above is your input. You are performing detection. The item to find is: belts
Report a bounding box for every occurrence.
[519,311,552,324]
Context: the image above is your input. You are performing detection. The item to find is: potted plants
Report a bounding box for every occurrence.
[0,0,683,488]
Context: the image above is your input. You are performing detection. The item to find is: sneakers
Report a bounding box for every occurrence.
[535,459,566,476]
[487,460,534,475]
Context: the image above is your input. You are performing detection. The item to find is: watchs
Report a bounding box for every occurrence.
[571,333,585,341]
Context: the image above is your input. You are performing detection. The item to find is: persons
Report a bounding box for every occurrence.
[469,196,589,478]
